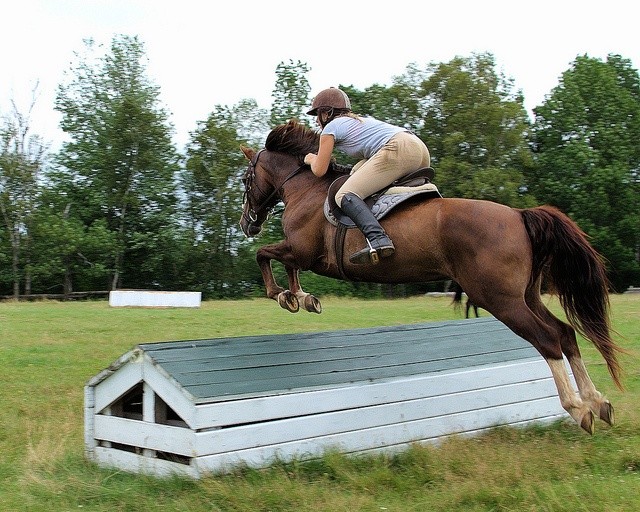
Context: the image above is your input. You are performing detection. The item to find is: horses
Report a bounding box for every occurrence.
[238,118,634,437]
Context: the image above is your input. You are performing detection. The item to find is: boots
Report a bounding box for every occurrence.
[341,192,396,264]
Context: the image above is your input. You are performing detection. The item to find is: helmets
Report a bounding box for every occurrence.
[306,87,351,117]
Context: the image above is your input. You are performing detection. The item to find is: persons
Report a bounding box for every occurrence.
[304,87,431,264]
